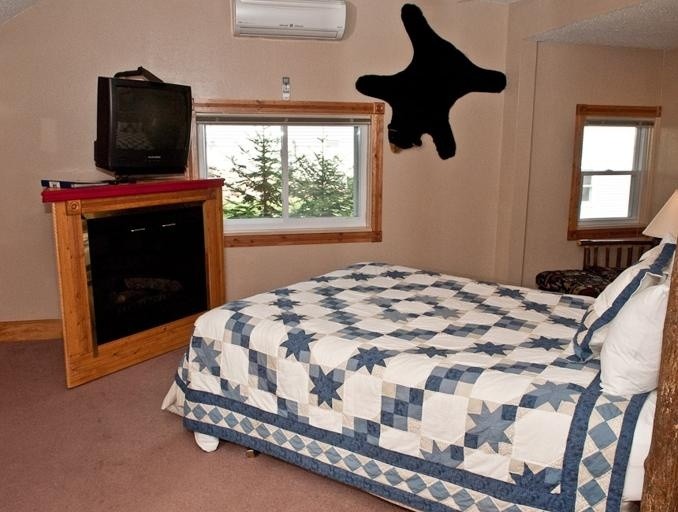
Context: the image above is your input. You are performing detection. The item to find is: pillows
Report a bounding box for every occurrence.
[570,232,675,362]
[599,284,668,397]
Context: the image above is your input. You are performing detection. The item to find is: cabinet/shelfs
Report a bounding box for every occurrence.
[48,173,227,392]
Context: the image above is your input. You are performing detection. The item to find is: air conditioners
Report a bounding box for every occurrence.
[229,0,347,41]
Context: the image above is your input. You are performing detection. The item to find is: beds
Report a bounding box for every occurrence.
[160,185,678,509]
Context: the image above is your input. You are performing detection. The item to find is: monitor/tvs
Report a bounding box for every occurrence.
[94,74,192,180]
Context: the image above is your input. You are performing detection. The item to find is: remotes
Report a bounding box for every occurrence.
[282,76,291,100]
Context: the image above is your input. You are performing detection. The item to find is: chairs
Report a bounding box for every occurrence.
[535,238,663,299]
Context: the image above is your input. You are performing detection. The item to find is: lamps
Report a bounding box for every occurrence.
[641,188,677,245]
